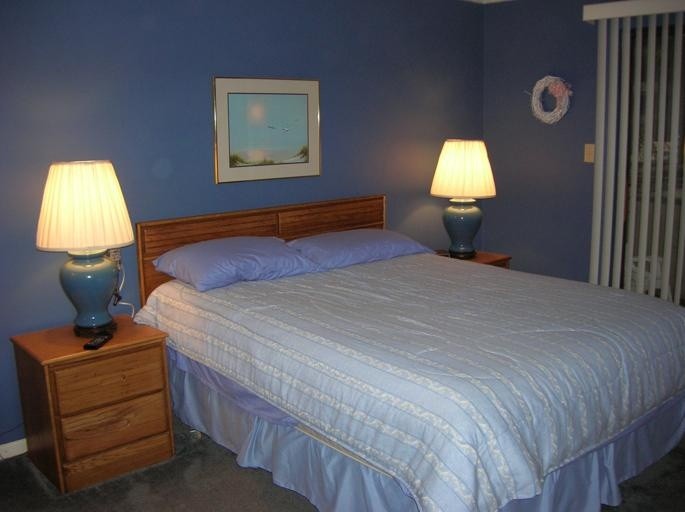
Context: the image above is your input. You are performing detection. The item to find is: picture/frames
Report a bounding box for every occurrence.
[211,74,321,185]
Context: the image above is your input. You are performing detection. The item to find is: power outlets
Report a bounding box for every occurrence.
[107,249,121,271]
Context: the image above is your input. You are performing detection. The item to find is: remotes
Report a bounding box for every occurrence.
[84,333,113,350]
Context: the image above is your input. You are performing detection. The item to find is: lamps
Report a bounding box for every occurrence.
[34,159,137,339]
[428,138,497,260]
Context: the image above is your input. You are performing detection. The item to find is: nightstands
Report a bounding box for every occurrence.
[432,249,512,270]
[8,311,176,498]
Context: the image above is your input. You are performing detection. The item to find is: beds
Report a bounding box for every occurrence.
[135,194,685,512]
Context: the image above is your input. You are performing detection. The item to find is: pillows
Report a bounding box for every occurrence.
[151,235,321,293]
[287,228,437,272]
